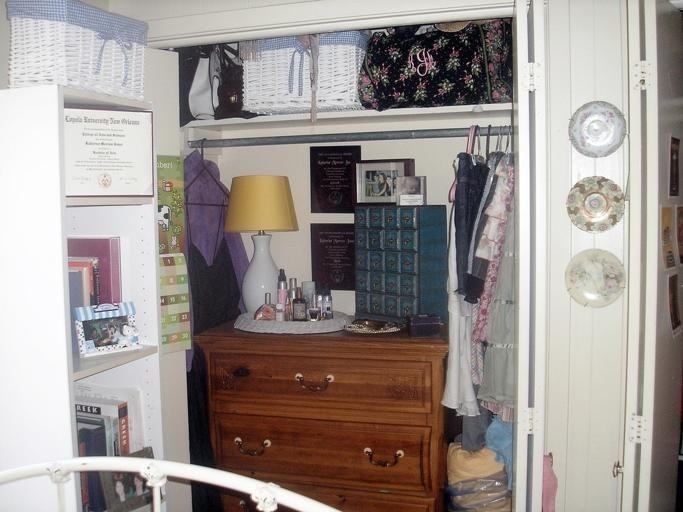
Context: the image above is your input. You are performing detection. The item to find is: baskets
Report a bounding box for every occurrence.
[239,30,371,115]
[7,0,148,101]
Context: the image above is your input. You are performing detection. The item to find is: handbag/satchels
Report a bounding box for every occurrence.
[357,18,513,111]
[189,43,267,120]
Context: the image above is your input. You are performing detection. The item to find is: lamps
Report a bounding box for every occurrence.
[221,175,297,319]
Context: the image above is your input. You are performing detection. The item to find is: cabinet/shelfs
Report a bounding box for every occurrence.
[0,82,167,512]
[190,316,447,511]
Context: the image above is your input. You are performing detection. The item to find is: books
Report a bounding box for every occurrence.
[75,395,130,512]
[68,256,102,353]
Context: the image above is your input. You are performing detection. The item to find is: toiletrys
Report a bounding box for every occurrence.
[254,268,333,322]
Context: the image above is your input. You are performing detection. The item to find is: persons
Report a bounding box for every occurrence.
[103,325,124,345]
[373,173,389,197]
[131,473,151,497]
[401,177,420,195]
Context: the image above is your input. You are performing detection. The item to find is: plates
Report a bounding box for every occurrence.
[565,177,625,233]
[570,100,626,158]
[565,249,627,308]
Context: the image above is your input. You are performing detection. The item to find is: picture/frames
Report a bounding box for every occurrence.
[351,159,413,205]
